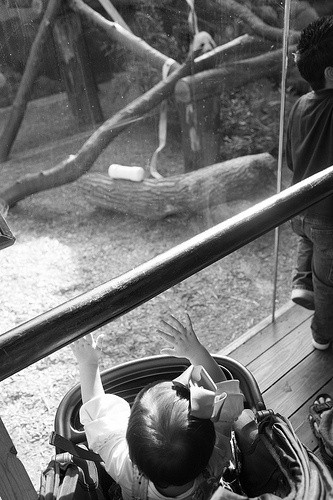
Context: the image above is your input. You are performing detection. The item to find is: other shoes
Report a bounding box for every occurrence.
[312,332,331,350]
[291,288,315,310]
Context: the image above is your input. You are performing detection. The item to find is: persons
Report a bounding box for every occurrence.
[70,312,245,500]
[309,392,333,462]
[285,19,333,350]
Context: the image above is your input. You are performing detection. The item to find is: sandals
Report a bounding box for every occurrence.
[308,392,333,467]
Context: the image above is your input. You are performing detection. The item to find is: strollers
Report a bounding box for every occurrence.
[39,355,333,500]
